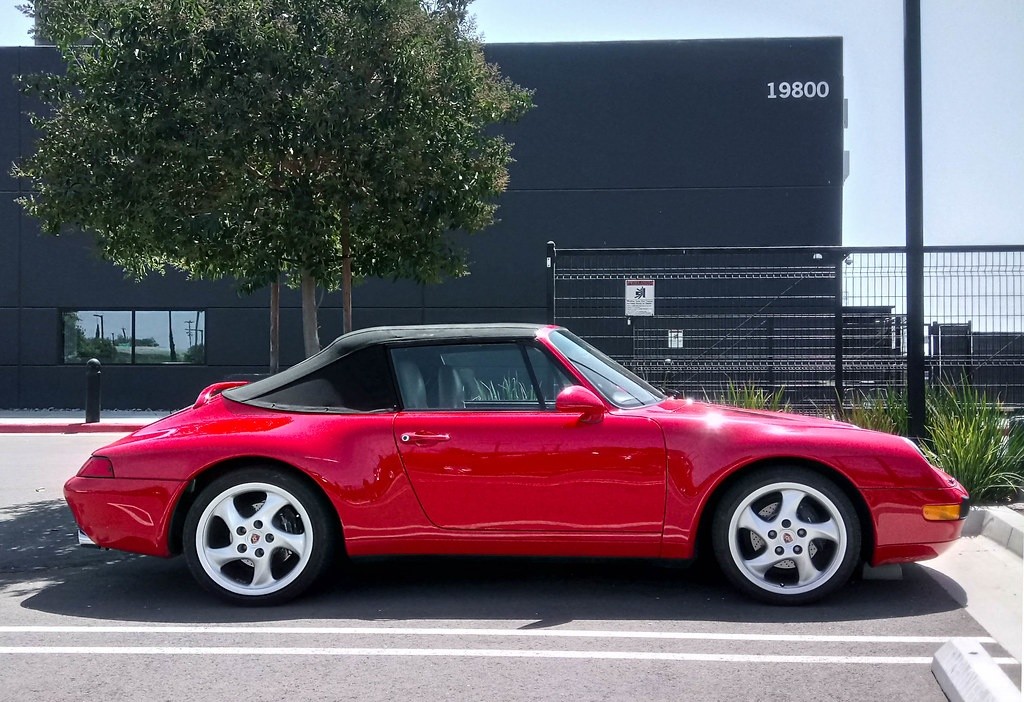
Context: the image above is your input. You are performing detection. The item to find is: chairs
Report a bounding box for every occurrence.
[397,360,428,408]
[438,365,465,408]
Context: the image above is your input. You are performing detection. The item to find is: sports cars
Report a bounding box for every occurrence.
[60,322,971,602]
